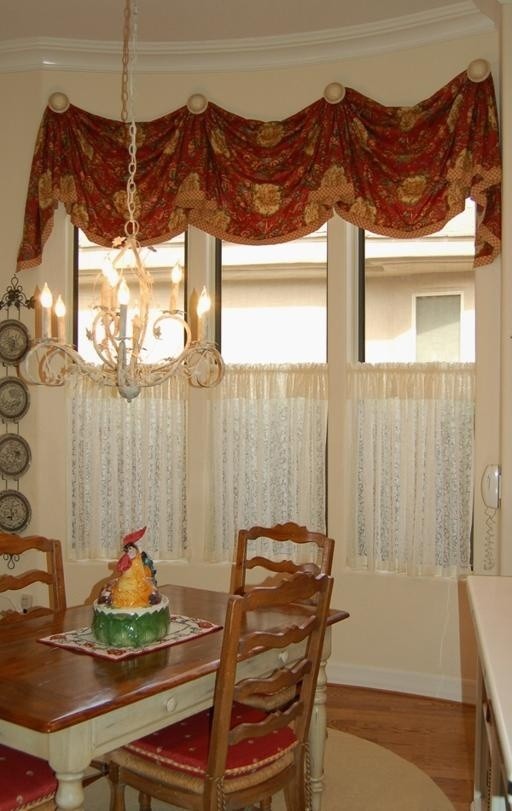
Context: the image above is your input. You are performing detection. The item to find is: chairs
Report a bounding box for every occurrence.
[1,745,60,811]
[2,528,68,614]
[229,522,337,808]
[102,571,335,808]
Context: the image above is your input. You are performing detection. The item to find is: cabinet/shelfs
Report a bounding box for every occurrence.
[465,575,511,811]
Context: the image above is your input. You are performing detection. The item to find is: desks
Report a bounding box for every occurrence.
[1,582,349,811]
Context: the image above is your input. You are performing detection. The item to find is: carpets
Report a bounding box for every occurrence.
[82,721,456,811]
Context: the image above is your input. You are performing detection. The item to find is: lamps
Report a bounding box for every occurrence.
[19,3,225,403]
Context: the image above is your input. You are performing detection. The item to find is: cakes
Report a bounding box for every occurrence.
[91,527,170,647]
